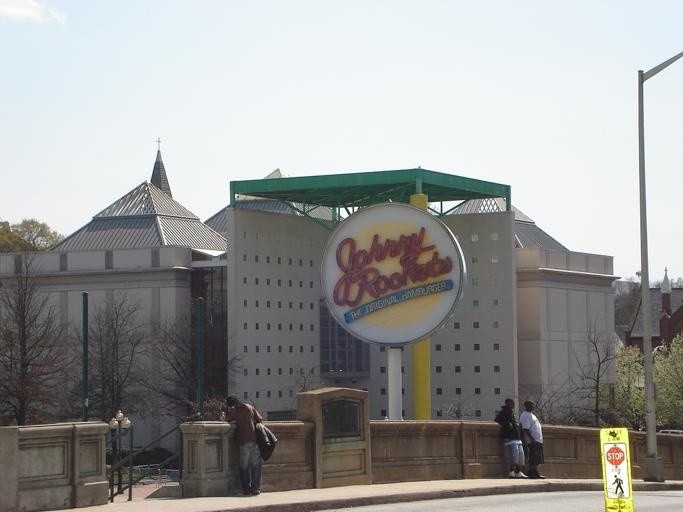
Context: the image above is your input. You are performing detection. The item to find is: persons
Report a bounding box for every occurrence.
[495,398,529,479]
[226,394,263,496]
[612,474,624,495]
[520,400,547,479]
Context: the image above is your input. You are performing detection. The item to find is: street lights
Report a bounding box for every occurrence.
[109,409,132,494]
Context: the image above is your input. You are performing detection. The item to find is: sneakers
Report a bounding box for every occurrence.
[530,469,545,478]
[243,489,260,496]
[516,470,528,478]
[509,470,515,478]
